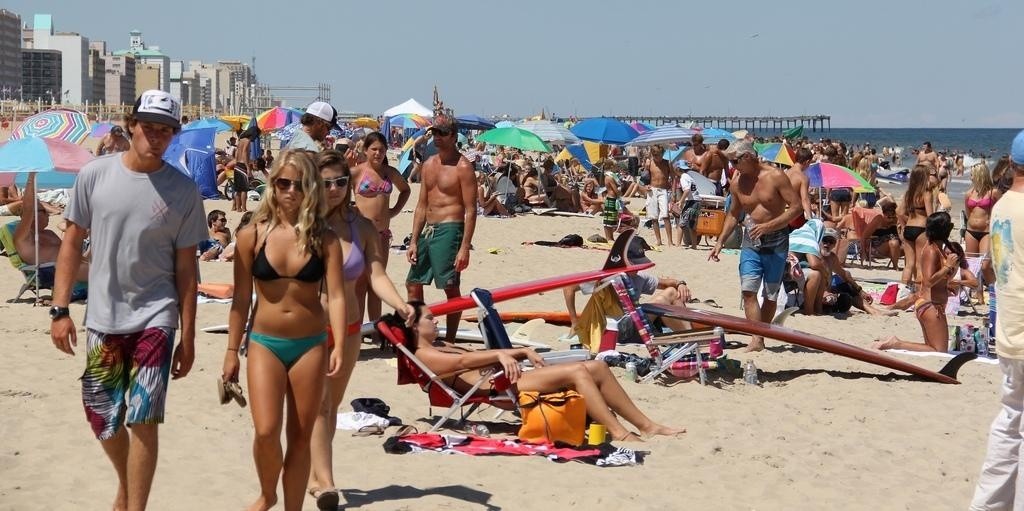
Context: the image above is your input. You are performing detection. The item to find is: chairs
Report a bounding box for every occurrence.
[947,252,987,309]
[483,157,497,176]
[0,220,88,307]
[469,287,593,370]
[536,166,559,208]
[852,207,907,268]
[608,272,724,389]
[378,320,520,436]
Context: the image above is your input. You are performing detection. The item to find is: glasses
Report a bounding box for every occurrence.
[276,178,303,191]
[823,237,835,244]
[323,176,349,187]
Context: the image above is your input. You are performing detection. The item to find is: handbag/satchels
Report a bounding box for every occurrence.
[640,170,651,184]
[516,389,586,447]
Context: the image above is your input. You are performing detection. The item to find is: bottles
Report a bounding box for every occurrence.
[960,324,989,357]
[741,211,762,249]
[742,358,760,385]
[462,421,490,437]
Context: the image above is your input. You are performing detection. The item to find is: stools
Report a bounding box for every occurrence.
[786,283,804,309]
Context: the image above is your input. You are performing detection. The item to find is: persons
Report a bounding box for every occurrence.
[0,88,1024,511]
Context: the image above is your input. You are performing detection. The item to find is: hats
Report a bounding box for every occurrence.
[307,102,343,132]
[428,115,457,136]
[133,90,181,131]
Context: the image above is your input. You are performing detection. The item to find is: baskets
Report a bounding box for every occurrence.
[696,208,725,236]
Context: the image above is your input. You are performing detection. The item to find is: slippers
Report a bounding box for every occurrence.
[317,488,339,511]
[393,426,426,436]
[217,374,246,406]
[352,425,384,437]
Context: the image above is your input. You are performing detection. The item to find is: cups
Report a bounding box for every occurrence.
[709,339,723,357]
[588,423,606,446]
